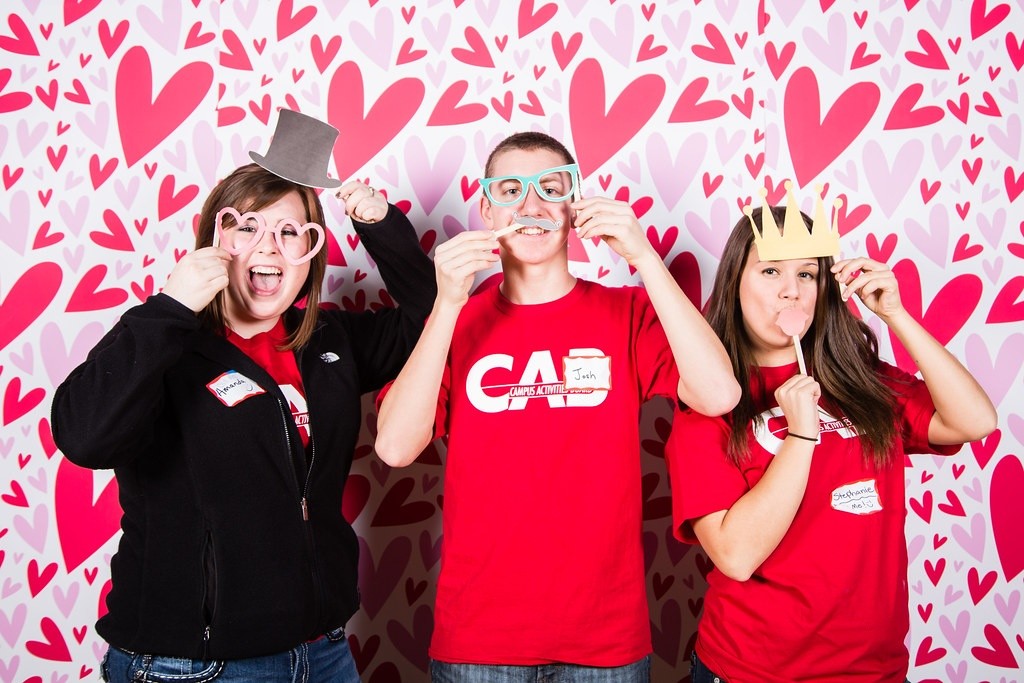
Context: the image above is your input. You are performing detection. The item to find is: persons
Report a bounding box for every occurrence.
[666,207,998,683]
[50,164,437,683]
[374,132,742,683]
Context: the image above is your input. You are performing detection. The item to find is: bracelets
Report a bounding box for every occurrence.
[788,432,818,441]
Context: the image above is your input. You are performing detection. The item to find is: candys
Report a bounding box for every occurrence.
[776,306,810,381]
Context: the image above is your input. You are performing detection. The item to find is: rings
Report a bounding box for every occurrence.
[369,187,374,197]
